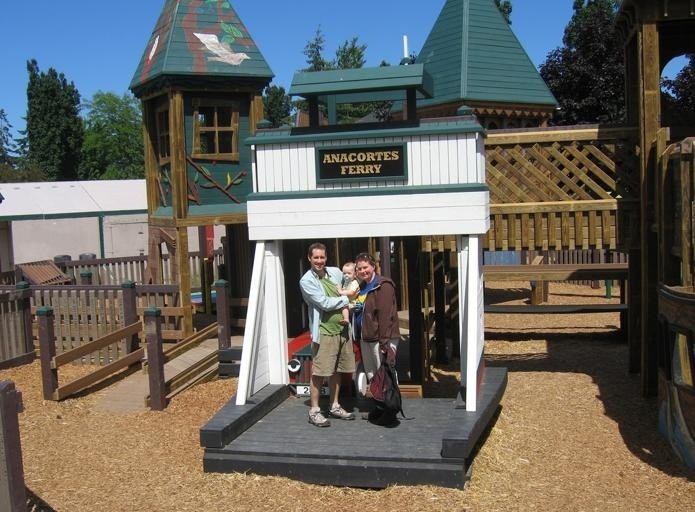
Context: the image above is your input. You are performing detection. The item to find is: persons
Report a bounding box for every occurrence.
[336,261,361,326]
[299,242,361,427]
[348,253,400,425]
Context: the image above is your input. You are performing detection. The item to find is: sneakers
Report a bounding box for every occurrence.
[327,404,354,420]
[362,407,384,419]
[308,407,330,427]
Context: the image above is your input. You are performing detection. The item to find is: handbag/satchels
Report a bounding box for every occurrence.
[369,362,401,414]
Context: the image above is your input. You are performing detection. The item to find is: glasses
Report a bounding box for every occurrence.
[356,254,370,259]
